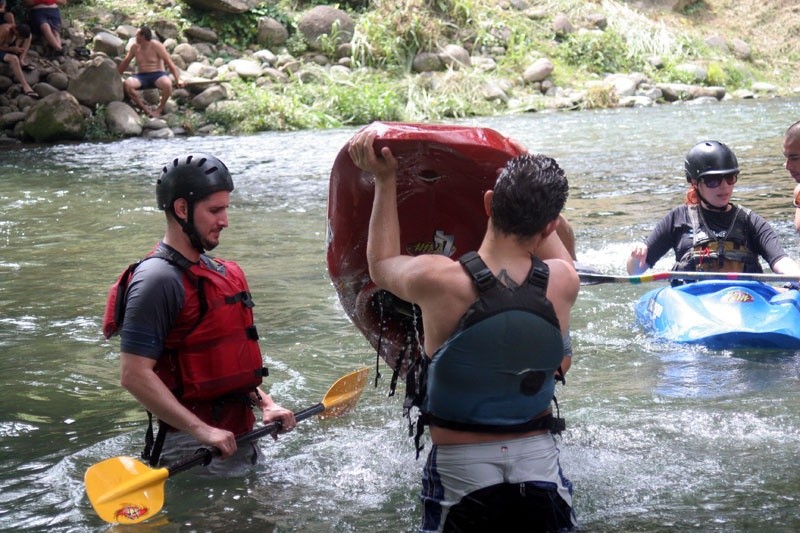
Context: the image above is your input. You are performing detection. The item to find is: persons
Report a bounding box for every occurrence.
[0,23,42,100]
[0,0,15,24]
[783,120,800,230]
[626,141,800,287]
[347,129,579,532]
[24,0,66,64]
[120,154,298,475]
[119,26,184,119]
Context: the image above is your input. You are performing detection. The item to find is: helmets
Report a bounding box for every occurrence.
[685,140,740,183]
[156,152,234,210]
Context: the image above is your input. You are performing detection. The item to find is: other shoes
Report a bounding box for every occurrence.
[22,64,33,70]
[25,91,39,97]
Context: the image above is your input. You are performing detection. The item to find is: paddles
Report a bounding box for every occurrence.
[572,267,800,285]
[84,367,369,525]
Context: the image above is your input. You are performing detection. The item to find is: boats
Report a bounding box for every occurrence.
[325,120,533,386]
[632,278,800,351]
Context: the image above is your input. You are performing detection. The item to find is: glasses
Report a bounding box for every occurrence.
[702,173,737,188]
